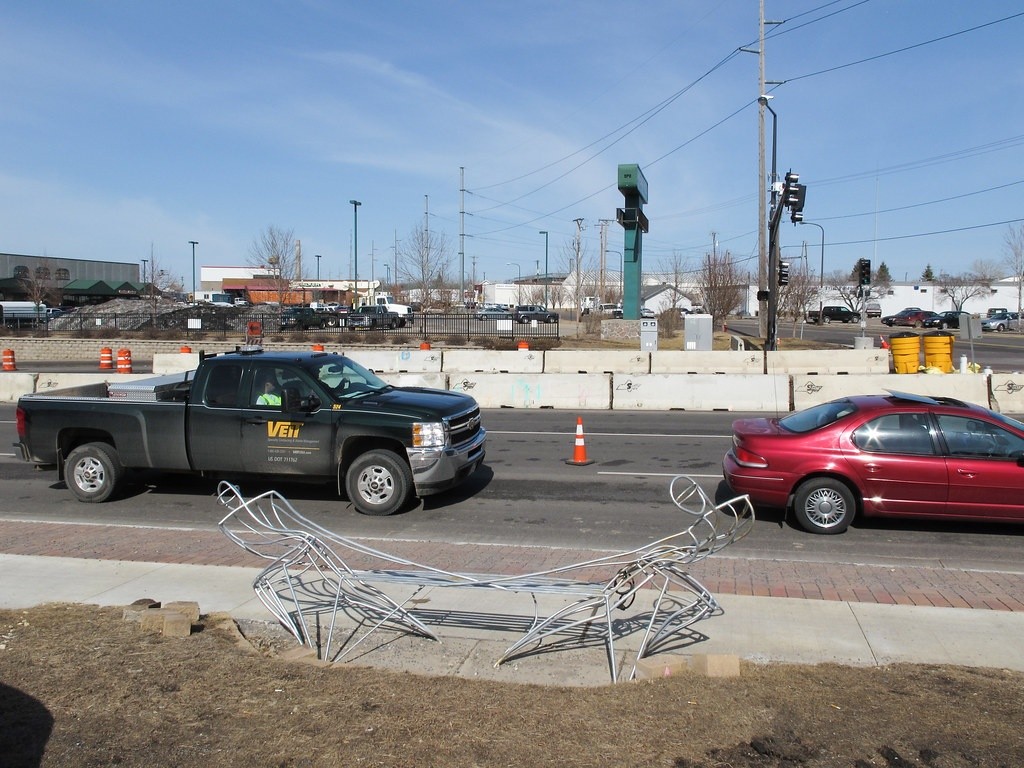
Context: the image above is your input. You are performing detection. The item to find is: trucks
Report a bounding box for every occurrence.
[212,293,250,308]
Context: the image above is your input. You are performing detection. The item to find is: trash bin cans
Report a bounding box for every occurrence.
[889,332,919,374]
[923,331,955,373]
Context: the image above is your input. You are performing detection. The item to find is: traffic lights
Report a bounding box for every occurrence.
[778,261,789,284]
[785,168,800,206]
[791,202,803,223]
[859,259,871,285]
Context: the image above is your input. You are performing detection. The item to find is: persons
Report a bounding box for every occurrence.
[250,368,282,406]
[295,365,348,399]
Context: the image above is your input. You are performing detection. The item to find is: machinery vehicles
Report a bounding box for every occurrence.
[0,301,63,329]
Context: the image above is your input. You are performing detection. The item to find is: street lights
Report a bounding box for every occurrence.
[188,240,199,305]
[507,263,521,305]
[350,199,363,312]
[539,231,548,308]
[141,259,149,283]
[799,221,825,325]
[759,95,778,352]
[605,250,622,296]
[315,254,322,280]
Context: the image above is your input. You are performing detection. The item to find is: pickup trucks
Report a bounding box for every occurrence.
[12,343,489,517]
[256,294,415,332]
[809,306,863,323]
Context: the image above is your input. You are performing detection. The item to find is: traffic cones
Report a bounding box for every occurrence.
[564,417,595,466]
[879,334,891,349]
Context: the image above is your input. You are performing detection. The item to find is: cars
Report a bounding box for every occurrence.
[981,311,1024,333]
[865,303,882,318]
[678,308,705,317]
[722,387,1024,536]
[581,295,623,319]
[882,307,938,328]
[922,311,971,330]
[641,308,656,318]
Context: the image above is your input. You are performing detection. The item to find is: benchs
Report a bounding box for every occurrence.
[216,474,759,686]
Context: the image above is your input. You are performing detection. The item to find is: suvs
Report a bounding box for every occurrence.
[424,298,560,324]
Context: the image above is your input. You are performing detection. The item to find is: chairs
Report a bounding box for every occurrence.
[902,414,930,448]
